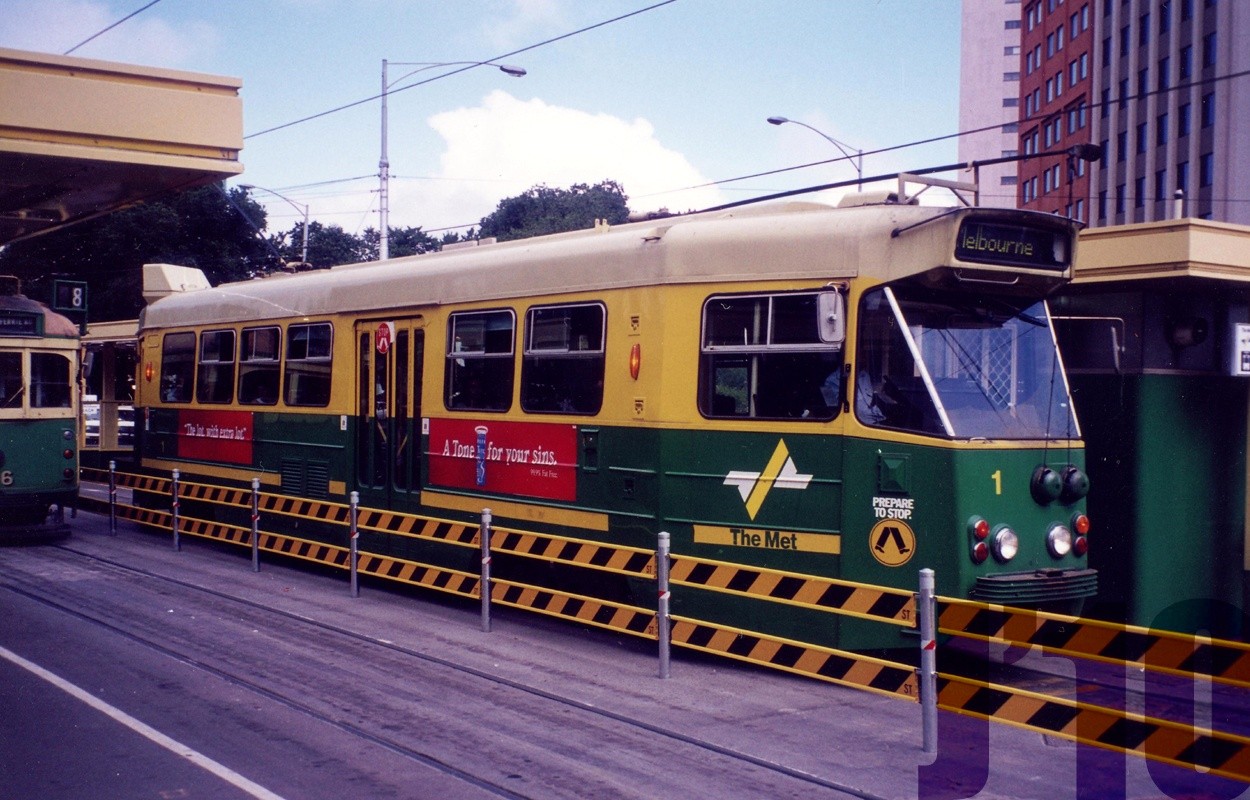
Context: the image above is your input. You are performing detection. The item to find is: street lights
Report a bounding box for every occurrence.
[765,115,864,196]
[374,55,531,258]
[236,181,310,263]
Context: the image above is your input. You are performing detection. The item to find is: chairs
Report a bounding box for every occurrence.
[242,367,329,408]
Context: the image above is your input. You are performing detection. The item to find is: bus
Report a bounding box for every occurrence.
[133,165,1104,658]
[0,273,85,548]
[1032,215,1250,677]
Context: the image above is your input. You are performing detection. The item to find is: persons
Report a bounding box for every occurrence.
[820,351,888,425]
